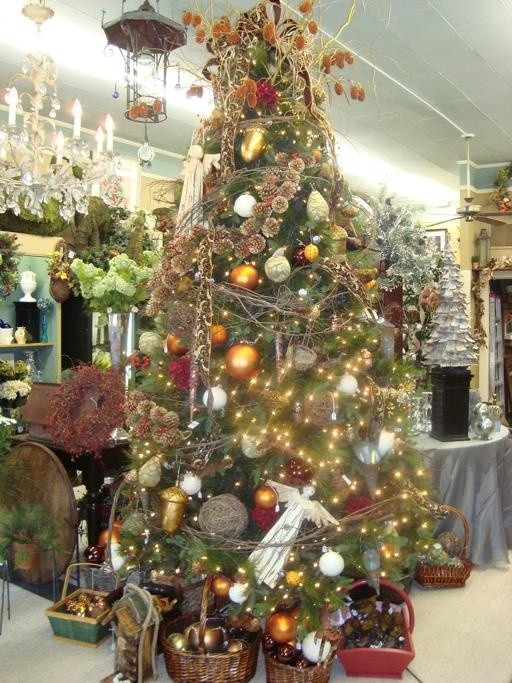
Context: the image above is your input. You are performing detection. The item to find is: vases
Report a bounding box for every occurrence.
[105,310,135,390]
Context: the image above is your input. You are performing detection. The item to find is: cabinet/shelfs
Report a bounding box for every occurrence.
[0,229,63,386]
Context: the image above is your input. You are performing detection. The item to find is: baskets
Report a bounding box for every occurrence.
[43,562,119,648]
[159,574,339,683]
[416,504,473,588]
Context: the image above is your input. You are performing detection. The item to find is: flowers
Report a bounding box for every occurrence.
[0,360,34,408]
[69,245,160,355]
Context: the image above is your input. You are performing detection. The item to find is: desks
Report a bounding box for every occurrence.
[408,424,511,565]
[18,409,131,555]
